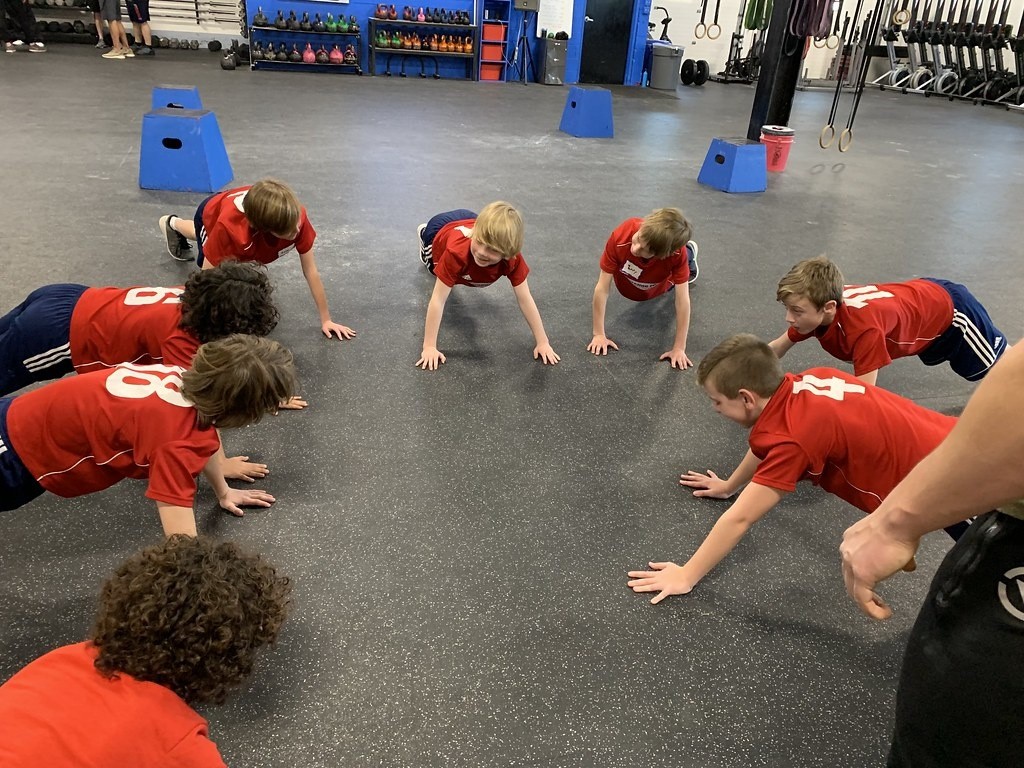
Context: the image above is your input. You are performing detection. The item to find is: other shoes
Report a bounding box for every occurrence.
[136,47,156,55]
[129,43,144,52]
[13,40,25,46]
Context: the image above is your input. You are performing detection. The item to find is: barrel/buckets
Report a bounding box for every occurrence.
[759,125,795,172]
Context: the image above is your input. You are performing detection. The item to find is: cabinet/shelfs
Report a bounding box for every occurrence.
[368,17,479,82]
[537,37,568,86]
[31,2,100,45]
[473,0,511,84]
[249,24,363,76]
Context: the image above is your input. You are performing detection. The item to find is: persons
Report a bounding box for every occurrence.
[159,177,356,340]
[586,206,699,370]
[0,0,47,52]
[125,0,155,55]
[0,533,294,768]
[840,336,1024,768]
[0,254,308,415]
[415,200,560,372]
[627,332,983,604]
[767,253,1013,387]
[86,0,135,59]
[0,332,301,538]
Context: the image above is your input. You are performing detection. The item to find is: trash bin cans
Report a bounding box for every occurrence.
[759,125,796,171]
[643,40,685,90]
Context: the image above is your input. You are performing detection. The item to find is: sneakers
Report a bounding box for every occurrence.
[686,240,699,283]
[417,223,427,266]
[5,42,17,53]
[101,46,126,59]
[29,43,47,53]
[121,49,135,57]
[94,41,107,49]
[159,215,196,261]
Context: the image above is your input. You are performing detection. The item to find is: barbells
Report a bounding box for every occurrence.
[681,59,709,86]
